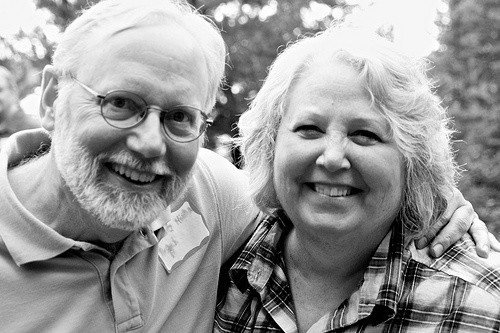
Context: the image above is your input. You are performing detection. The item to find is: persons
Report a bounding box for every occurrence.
[213,20,500,332]
[0,0,499,332]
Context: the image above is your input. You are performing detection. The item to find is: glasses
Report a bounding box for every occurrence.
[59,73,216,143]
